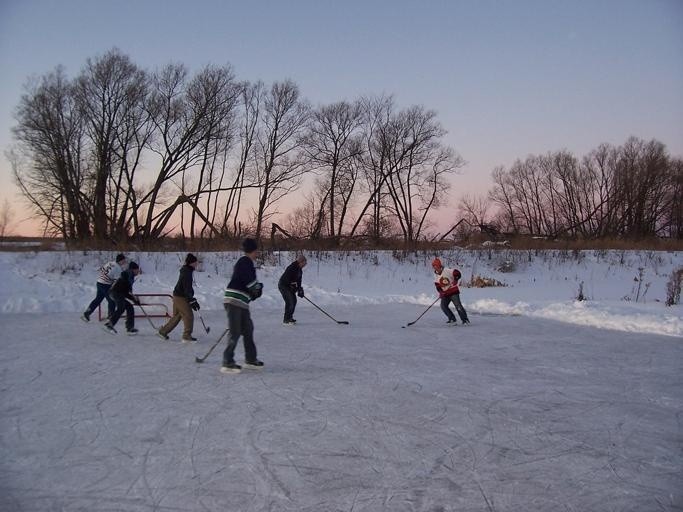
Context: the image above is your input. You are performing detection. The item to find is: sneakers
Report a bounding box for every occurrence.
[183,337,197,341]
[284,318,296,323]
[246,360,263,366]
[159,331,168,339]
[224,364,241,369]
[447,318,457,323]
[461,319,470,324]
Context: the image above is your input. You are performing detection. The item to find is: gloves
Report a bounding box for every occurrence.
[249,289,263,301]
[297,287,304,298]
[189,298,200,311]
[452,280,457,286]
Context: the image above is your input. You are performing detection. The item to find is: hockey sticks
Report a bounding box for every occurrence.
[401,297,442,328]
[304,295,349,325]
[196,308,210,334]
[195,329,229,363]
[138,302,159,330]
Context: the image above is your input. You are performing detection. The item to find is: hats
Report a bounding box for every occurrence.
[243,238,257,253]
[116,254,125,262]
[129,262,139,269]
[297,255,305,263]
[185,253,197,265]
[432,259,441,269]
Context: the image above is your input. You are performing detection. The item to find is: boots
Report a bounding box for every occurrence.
[104,319,116,333]
[84,308,92,320]
[127,327,137,332]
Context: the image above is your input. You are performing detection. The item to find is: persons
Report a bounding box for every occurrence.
[277,255,307,324]
[83,253,125,321]
[222,239,263,369]
[432,259,468,324]
[105,261,139,333]
[158,253,197,341]
